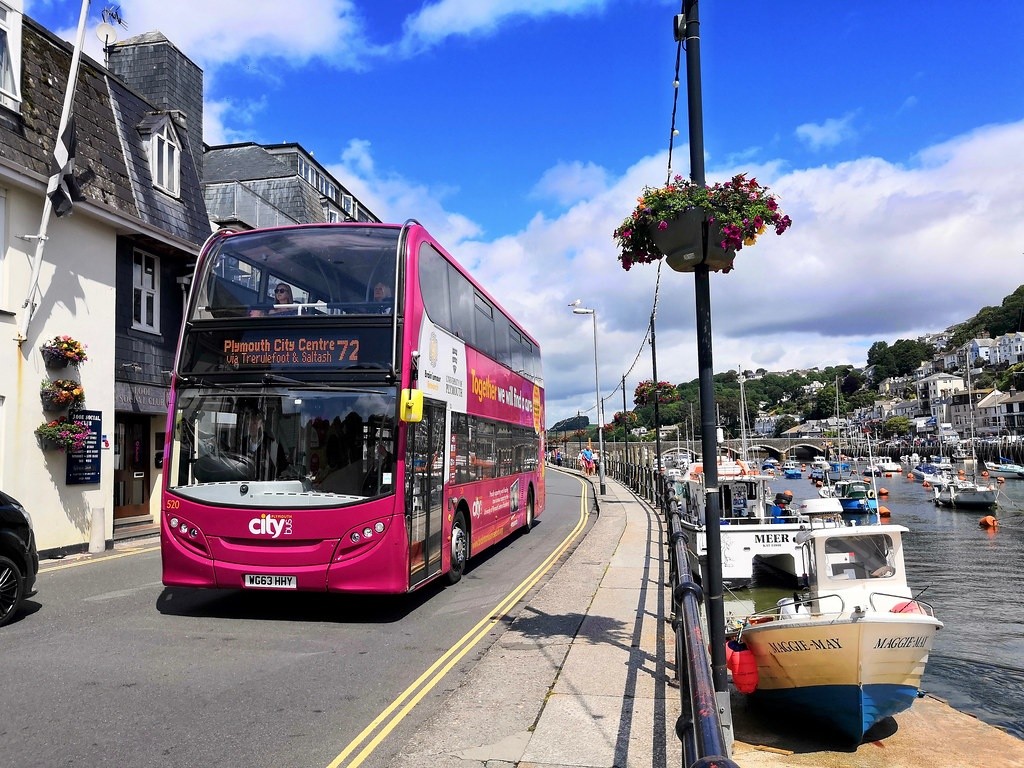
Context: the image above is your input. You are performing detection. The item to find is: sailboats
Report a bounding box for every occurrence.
[927,350,1001,508]
[809,375,852,484]
[983,383,1024,481]
[784,420,802,480]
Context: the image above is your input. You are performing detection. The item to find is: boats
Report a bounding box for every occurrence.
[911,459,951,487]
[818,444,870,500]
[910,453,919,462]
[763,464,774,474]
[735,382,943,749]
[811,453,830,471]
[862,464,882,477]
[779,460,793,472]
[875,455,901,472]
[624,362,827,592]
[951,444,970,463]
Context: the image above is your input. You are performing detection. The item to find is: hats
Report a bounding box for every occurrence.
[784,491,792,495]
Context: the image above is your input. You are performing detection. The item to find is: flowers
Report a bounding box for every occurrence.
[615,168,793,272]
[36,416,91,456]
[44,335,87,371]
[40,375,86,412]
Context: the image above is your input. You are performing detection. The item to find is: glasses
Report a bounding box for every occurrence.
[274,288,286,293]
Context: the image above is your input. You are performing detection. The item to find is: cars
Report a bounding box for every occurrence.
[0,492,40,626]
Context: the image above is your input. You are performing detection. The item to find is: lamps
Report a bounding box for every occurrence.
[123,362,142,374]
[160,368,174,380]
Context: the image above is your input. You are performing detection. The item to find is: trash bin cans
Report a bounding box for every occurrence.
[114,480,126,506]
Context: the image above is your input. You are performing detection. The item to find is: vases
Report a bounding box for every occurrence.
[44,350,68,369]
[42,393,71,412]
[39,433,58,451]
[647,206,736,271]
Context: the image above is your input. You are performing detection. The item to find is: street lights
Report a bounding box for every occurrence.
[574,308,606,494]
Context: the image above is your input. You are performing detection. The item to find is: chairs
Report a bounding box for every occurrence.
[244,409,290,455]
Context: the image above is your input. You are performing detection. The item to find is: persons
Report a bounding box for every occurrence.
[368,282,393,314]
[557,452,561,467]
[238,410,287,481]
[582,446,594,476]
[770,503,785,524]
[269,283,305,315]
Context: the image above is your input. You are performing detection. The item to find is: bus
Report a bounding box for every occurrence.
[158,218,546,596]
